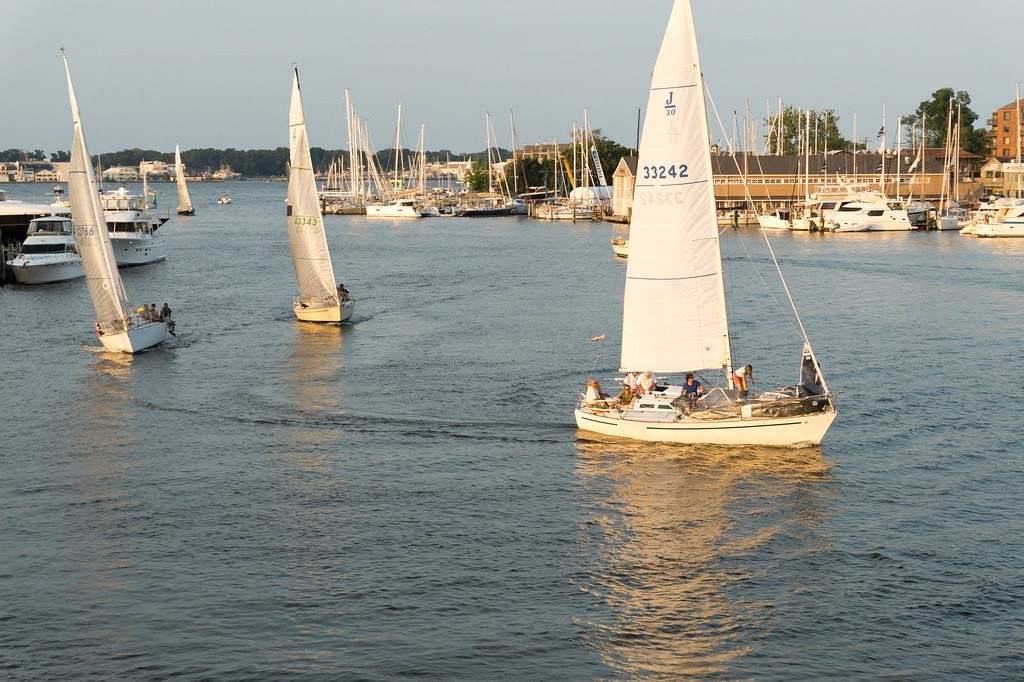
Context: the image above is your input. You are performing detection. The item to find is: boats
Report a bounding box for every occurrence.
[54,186,63,194]
[217,196,232,205]
[6,213,86,285]
[99,187,166,268]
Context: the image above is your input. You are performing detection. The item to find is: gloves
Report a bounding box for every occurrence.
[751,380,754,384]
[744,390,748,396]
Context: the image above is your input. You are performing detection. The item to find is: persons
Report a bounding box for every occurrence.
[336,283,350,302]
[585,370,658,412]
[139,303,176,337]
[732,365,755,399]
[679,372,704,409]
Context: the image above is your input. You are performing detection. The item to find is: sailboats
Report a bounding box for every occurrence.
[59,46,167,356]
[317,90,1024,239]
[96,154,104,196]
[285,62,356,323]
[573,0,841,449]
[174,144,196,217]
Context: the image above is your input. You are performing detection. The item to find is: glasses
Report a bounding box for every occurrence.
[594,382,597,384]
[690,376,693,379]
[625,389,629,390]
